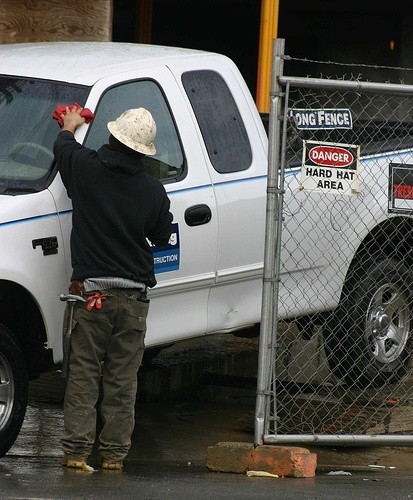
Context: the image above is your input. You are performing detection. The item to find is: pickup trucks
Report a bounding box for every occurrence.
[0,42,413,460]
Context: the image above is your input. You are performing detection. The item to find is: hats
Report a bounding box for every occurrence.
[107,107,156,155]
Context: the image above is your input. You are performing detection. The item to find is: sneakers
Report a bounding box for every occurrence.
[101,460,123,469]
[61,454,86,469]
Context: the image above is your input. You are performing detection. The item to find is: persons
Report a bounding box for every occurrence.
[53,106,174,471]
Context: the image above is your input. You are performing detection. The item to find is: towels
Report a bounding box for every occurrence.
[51,102,95,126]
